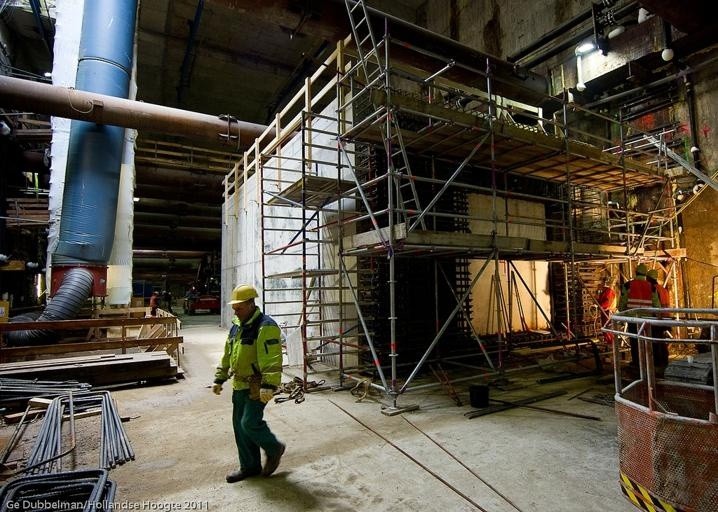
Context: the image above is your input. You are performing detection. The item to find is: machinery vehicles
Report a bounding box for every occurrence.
[181,252,221,317]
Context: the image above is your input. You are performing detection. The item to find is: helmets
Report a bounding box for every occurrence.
[227,284,259,305]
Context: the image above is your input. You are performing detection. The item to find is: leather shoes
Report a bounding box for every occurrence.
[264,444,285,476]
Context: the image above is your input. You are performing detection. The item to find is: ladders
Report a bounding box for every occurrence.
[345,0,427,231]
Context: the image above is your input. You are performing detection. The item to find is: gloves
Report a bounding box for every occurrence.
[225,466,262,483]
[260,389,274,404]
[211,383,224,396]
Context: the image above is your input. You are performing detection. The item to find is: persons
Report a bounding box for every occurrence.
[646,269,669,357]
[617,263,660,369]
[212,284,285,483]
[593,276,616,352]
[150,290,173,315]
